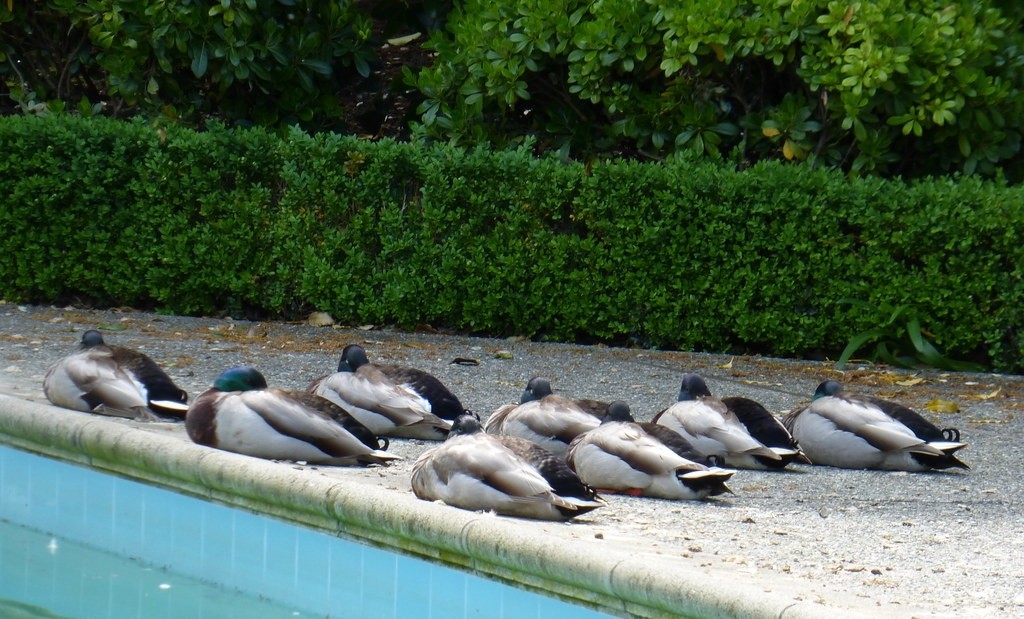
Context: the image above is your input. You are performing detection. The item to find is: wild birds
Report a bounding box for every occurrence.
[652,374,813,472]
[185,366,403,467]
[566,401,735,499]
[410,411,609,520]
[305,344,481,441]
[783,382,972,472]
[485,377,610,464]
[44,329,189,423]
